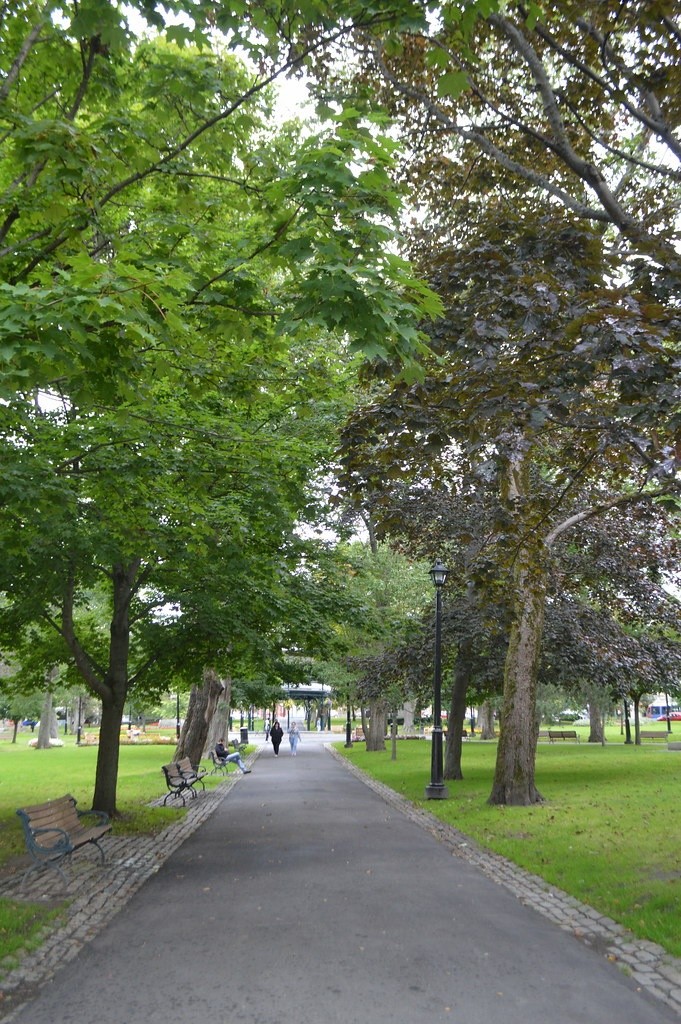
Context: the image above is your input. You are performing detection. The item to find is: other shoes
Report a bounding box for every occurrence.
[265,741,269,743]
[275,754,278,758]
[291,752,296,756]
[244,770,251,774]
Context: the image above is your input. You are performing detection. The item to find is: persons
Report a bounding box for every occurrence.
[316,718,321,732]
[270,722,284,757]
[287,722,301,756]
[264,718,270,741]
[272,719,281,727]
[215,738,252,775]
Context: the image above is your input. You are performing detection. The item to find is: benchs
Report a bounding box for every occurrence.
[126,730,141,739]
[538,730,549,740]
[548,731,581,744]
[211,749,230,776]
[160,758,209,806]
[16,792,114,897]
[351,726,365,740]
[231,739,247,756]
[640,731,669,745]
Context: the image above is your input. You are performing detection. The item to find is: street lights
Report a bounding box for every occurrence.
[426,559,451,799]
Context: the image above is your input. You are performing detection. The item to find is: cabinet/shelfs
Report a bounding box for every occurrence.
[444,730,469,741]
